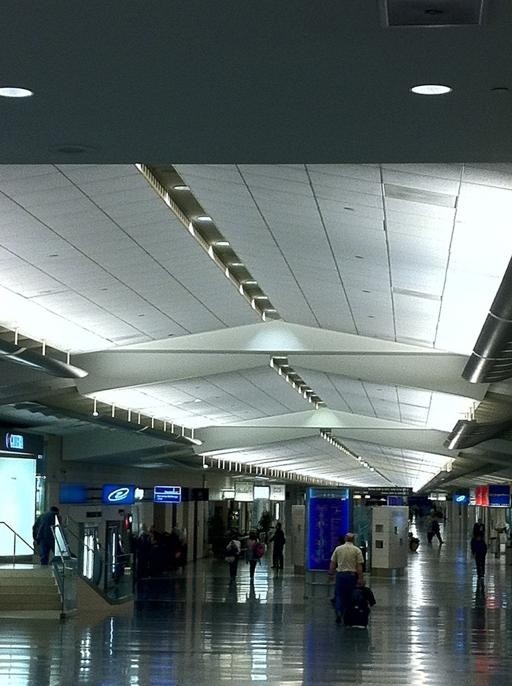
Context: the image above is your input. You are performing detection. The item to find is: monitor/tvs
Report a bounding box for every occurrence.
[102,483,138,505]
[152,484,182,505]
[453,494,469,505]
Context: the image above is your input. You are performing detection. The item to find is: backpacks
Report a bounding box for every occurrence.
[253,541,265,559]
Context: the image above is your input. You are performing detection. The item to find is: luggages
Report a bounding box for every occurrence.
[342,586,376,627]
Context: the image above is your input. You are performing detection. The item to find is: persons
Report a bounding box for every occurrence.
[330,532,364,623]
[474,518,485,538]
[408,502,444,551]
[471,534,487,577]
[136,529,186,577]
[246,532,262,579]
[267,523,285,569]
[32,507,62,565]
[225,535,241,585]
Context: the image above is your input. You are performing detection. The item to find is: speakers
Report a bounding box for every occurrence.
[182,487,189,502]
[192,488,209,501]
